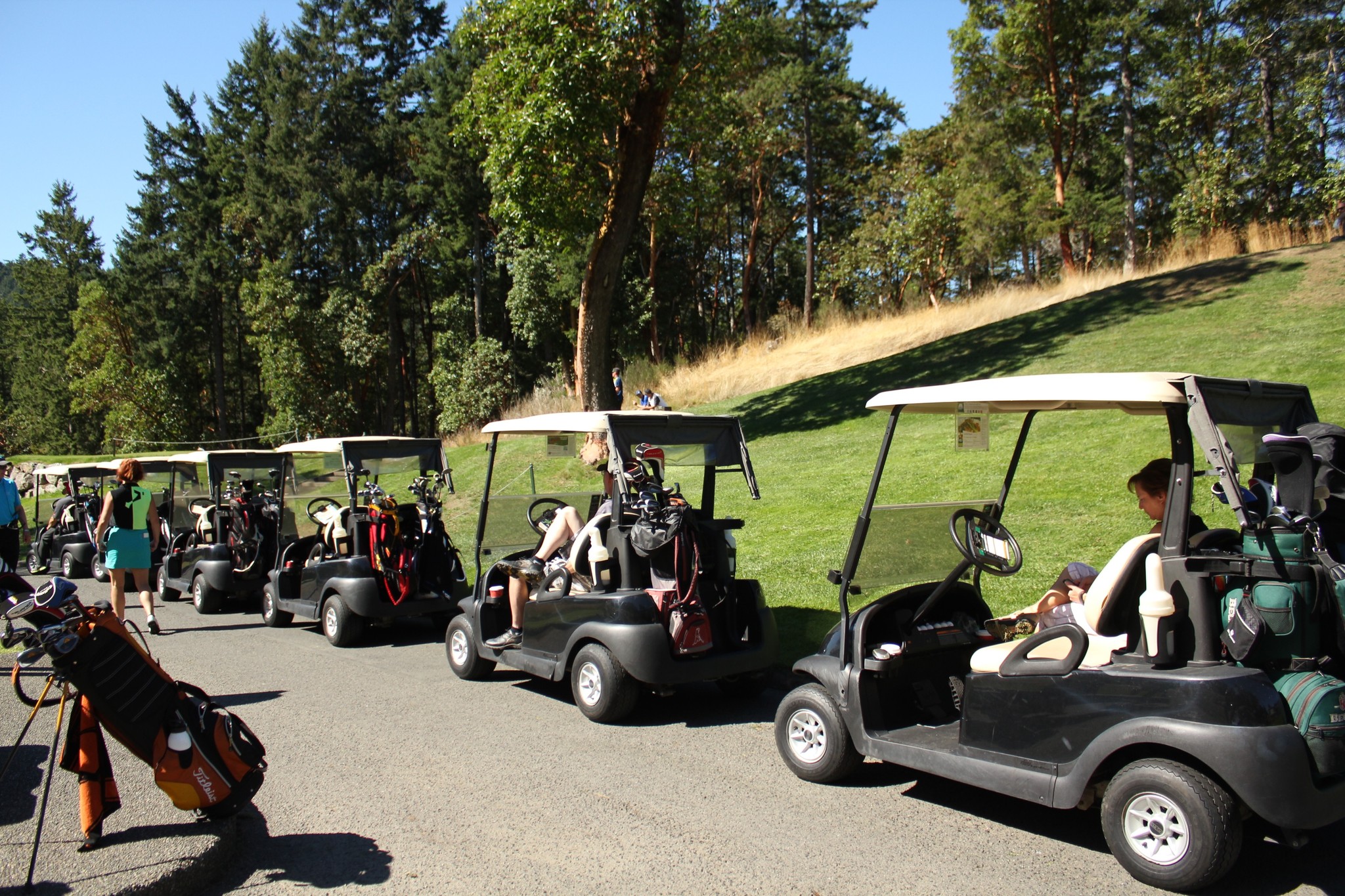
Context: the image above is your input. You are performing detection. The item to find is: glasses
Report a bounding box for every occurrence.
[601,470,605,476]
[0,465,7,470]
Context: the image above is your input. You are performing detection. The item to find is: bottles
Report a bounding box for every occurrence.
[166,722,193,769]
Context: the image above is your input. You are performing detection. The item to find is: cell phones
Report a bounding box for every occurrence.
[1063,578,1077,586]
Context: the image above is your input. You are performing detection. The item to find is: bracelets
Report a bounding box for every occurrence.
[23,528,29,532]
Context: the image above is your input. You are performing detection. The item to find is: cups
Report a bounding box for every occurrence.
[489,585,504,598]
[174,548,180,553]
[881,644,902,656]
[976,630,994,640]
[286,560,293,568]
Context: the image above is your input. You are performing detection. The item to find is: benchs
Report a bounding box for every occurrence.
[191,504,230,548]
[54,503,86,538]
[551,510,640,593]
[304,504,371,567]
[970,533,1163,673]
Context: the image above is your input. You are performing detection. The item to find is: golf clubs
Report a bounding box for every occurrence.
[629,483,675,527]
[0,618,79,668]
[223,469,280,505]
[360,466,453,510]
[78,479,119,498]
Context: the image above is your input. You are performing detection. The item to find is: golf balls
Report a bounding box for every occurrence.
[1246,505,1312,525]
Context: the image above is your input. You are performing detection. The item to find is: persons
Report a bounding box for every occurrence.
[96,458,162,635]
[483,457,633,650]
[30,480,80,573]
[0,455,32,574]
[611,367,623,410]
[981,458,1210,636]
[632,389,669,411]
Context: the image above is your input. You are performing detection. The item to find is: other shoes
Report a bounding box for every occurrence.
[983,616,1035,639]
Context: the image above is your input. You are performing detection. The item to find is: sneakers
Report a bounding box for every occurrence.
[484,628,524,650]
[496,556,545,583]
[147,615,161,635]
[31,565,46,573]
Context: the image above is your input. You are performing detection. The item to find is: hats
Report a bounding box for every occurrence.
[0,455,12,466]
[636,390,642,395]
[63,482,71,492]
[596,460,609,472]
